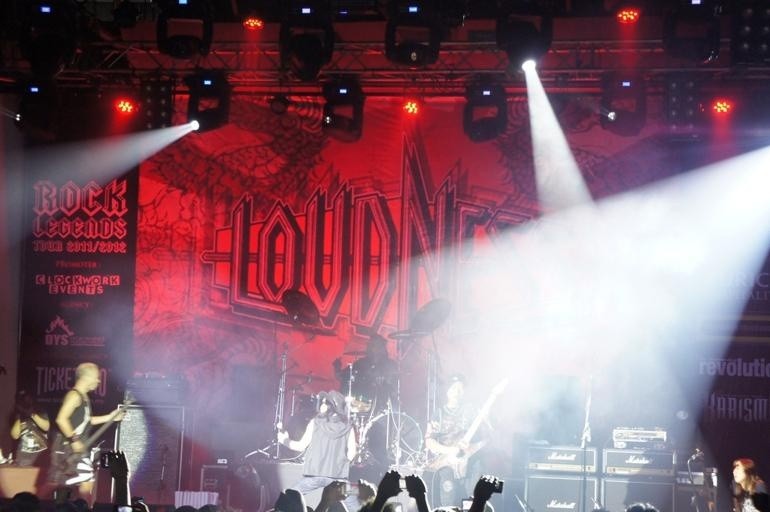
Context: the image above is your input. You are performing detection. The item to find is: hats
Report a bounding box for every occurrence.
[319,390,348,416]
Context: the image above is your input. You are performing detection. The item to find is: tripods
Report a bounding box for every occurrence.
[241,354,302,462]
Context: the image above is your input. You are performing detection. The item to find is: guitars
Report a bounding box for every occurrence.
[50,393,135,486]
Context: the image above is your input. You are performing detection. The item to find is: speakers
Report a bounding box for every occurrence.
[111,405,186,506]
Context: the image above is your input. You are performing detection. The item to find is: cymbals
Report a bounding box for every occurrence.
[386,328,429,343]
[410,299,450,340]
[282,289,320,331]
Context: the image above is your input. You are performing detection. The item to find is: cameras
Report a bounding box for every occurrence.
[100,453,118,469]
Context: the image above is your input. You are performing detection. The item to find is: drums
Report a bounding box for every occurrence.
[342,398,374,420]
[362,411,424,469]
[298,393,322,416]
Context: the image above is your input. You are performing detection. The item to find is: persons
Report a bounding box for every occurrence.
[276,390,358,498]
[5,450,224,511]
[272,470,500,511]
[46,363,127,497]
[332,334,398,432]
[423,376,495,507]
[624,458,768,511]
[9,388,51,466]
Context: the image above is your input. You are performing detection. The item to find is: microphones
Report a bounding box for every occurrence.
[687,448,705,464]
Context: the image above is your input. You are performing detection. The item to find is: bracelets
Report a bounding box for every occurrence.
[283,438,292,448]
[66,434,81,445]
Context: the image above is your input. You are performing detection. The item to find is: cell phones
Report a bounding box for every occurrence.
[398,478,407,489]
[494,480,504,493]
[135,497,144,505]
[342,483,360,496]
